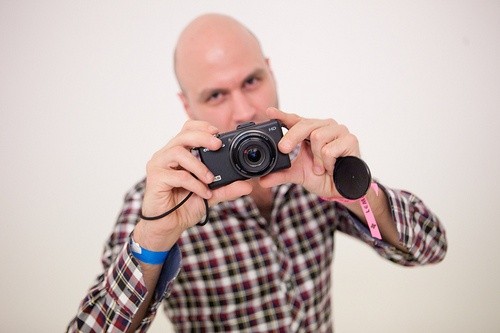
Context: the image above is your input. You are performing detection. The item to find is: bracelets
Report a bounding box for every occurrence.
[128,234,175,266]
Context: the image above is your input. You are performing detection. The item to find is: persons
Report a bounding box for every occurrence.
[67,13,448,333]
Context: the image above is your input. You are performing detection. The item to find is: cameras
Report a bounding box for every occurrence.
[197,119,292,190]
